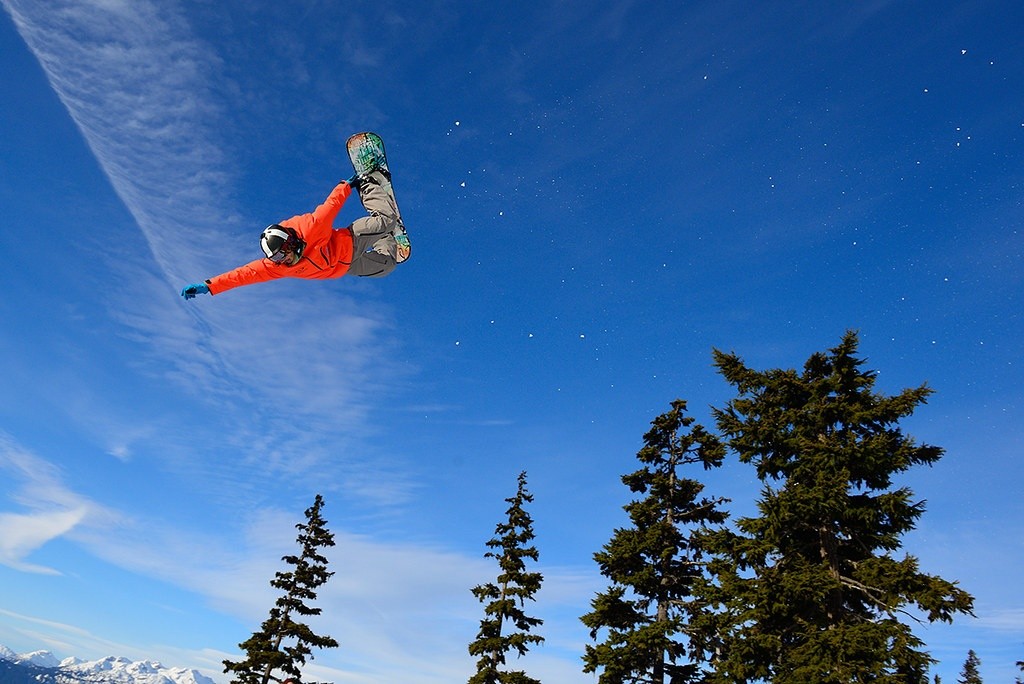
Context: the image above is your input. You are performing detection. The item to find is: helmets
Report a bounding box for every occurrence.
[260,224,289,259]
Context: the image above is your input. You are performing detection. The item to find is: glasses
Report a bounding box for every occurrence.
[270,249,285,264]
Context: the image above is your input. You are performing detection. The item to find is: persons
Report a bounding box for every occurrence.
[180,175,398,300]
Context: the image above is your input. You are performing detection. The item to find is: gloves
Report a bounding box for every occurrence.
[181,283,208,300]
[347,175,359,188]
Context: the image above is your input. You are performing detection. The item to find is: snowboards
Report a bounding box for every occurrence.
[346,131,412,266]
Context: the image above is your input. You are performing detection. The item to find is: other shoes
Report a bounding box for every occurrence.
[354,176,379,196]
